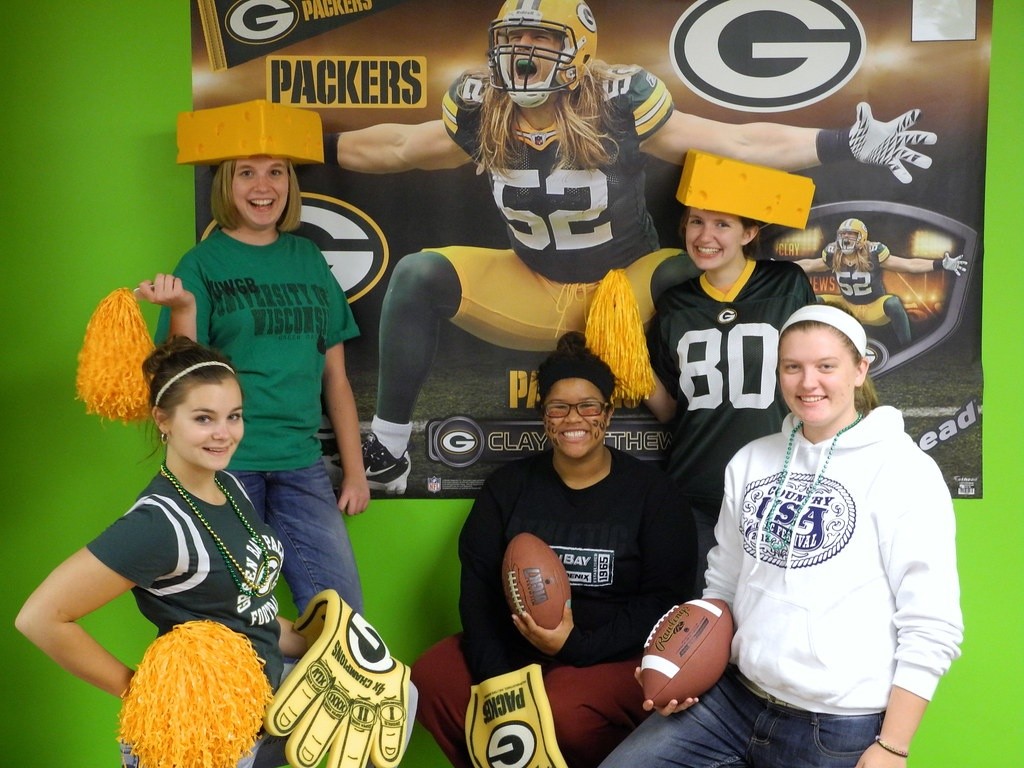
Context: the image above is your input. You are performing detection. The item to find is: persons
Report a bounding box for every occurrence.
[638,208,815,599]
[595,301,964,768]
[792,218,966,347]
[15,336,418,768]
[407,333,699,768]
[138,155,371,614]
[289,0,936,491]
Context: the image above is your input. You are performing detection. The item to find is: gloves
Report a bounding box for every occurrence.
[464,664,568,768]
[263,588,417,768]
[815,101,937,186]
[932,251,968,276]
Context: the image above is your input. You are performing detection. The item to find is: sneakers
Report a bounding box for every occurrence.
[322,433,410,494]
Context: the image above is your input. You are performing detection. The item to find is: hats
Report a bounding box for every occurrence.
[536,331,615,400]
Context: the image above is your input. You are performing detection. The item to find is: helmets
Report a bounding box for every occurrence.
[837,219,867,255]
[490,0,596,107]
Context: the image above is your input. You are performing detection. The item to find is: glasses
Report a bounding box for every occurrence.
[545,399,608,419]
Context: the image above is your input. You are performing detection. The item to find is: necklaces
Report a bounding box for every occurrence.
[766,411,862,549]
[160,462,269,596]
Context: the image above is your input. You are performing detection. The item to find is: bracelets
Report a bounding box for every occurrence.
[875,735,908,757]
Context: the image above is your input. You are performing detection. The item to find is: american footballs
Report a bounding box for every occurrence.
[501,532,571,630]
[640,599,734,708]
